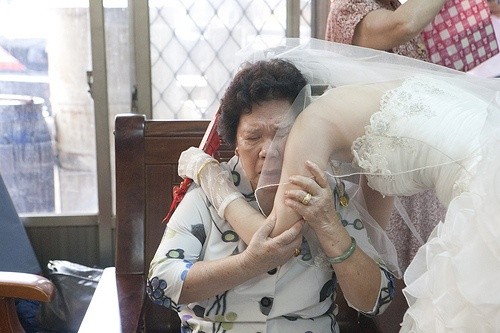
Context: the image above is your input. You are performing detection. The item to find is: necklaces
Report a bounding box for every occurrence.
[328,160,350,208]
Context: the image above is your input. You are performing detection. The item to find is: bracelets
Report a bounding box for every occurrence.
[196,160,220,187]
[326,236,356,264]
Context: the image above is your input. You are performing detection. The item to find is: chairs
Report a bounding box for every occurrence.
[77,113,213,332]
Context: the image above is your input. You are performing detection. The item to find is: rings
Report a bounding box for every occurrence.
[302,193,311,204]
[294,248,301,257]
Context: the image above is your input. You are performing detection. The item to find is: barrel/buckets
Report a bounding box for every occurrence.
[58,166,98,214]
[57,5,135,92]
[0,94,56,215]
[50,90,131,171]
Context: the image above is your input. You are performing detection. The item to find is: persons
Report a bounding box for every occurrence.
[178,43,500,333]
[145,59,394,333]
[326,1,500,333]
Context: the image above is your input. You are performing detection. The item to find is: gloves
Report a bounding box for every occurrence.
[177,146,220,184]
